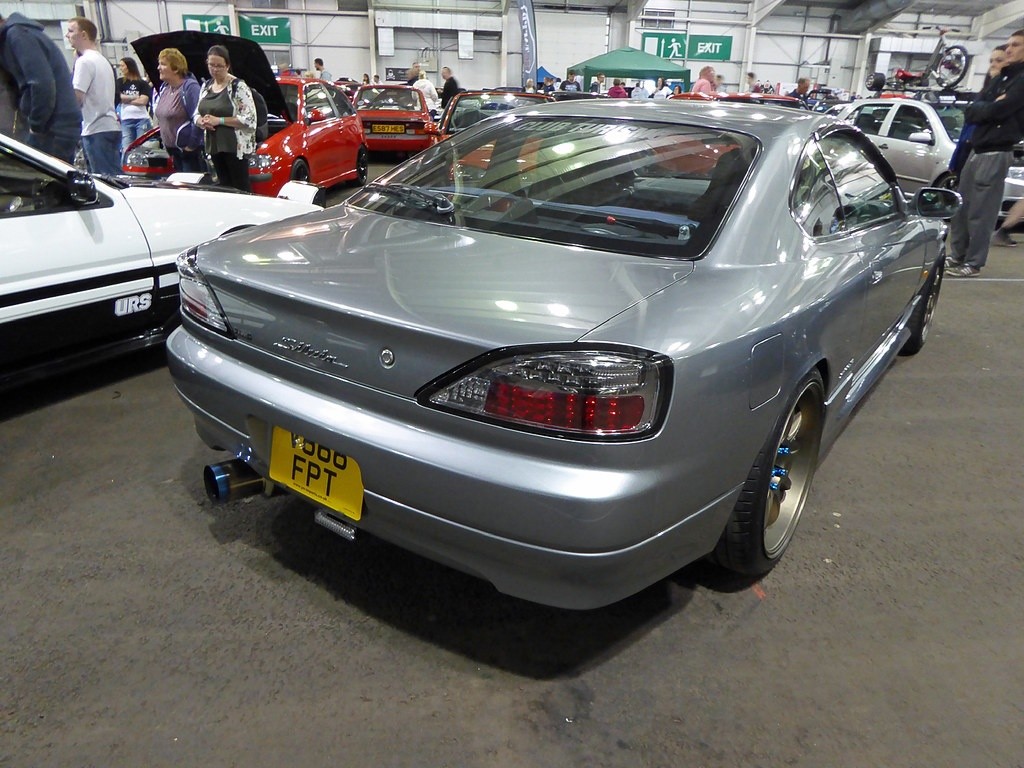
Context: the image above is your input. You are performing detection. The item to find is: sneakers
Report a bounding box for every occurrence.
[946,264,980,277]
[944,256,961,269]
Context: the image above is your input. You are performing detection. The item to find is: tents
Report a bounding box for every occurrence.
[567,46,691,92]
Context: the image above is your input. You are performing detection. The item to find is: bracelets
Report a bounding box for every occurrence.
[219,117,224,126]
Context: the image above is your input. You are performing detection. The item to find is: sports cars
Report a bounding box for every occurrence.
[166,96,964,611]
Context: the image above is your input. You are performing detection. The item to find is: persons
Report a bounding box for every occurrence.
[559,70,581,92]
[608,78,628,98]
[296,58,332,82]
[942,29,1024,277]
[540,77,555,92]
[589,72,605,93]
[630,83,649,98]
[689,66,722,93]
[649,77,681,99]
[0,12,257,192]
[746,73,761,93]
[521,78,536,92]
[406,62,459,119]
[362,74,382,85]
[782,78,810,110]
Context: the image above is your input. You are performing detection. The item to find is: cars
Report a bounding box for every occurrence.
[334,76,434,163]
[434,90,552,212]
[0,129,329,400]
[123,29,371,207]
[556,90,1024,227]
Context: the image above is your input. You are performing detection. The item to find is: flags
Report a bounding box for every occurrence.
[517,0,537,85]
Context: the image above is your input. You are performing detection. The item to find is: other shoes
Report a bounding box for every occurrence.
[991,235,1017,246]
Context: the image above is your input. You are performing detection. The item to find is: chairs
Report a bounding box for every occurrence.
[940,116,960,139]
[687,148,755,222]
[895,112,918,139]
[396,95,415,107]
[532,136,639,207]
[857,113,878,135]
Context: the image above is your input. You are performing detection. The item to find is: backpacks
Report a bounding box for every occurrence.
[204,77,268,127]
